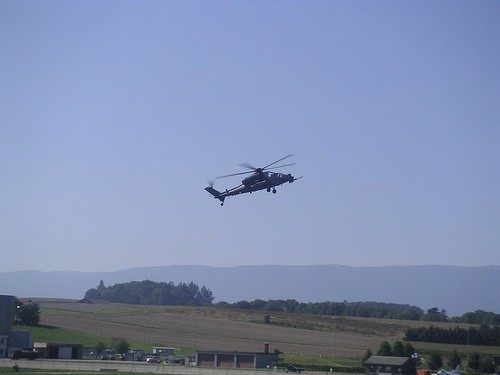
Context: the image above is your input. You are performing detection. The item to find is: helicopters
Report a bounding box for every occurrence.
[204,153,304,206]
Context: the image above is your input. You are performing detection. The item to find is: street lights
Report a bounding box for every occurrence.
[194,310,200,348]
[459,326,470,358]
[144,310,148,352]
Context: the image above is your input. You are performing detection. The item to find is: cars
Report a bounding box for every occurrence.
[88,348,149,362]
[145,355,161,363]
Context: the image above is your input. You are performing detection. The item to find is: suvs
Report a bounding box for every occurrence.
[152,346,185,366]
